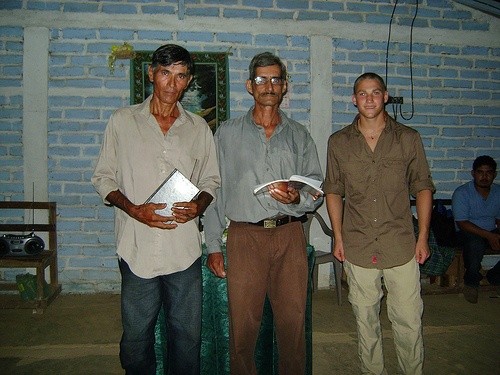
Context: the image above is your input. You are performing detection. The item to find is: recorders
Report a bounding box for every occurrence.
[0,182,46,256]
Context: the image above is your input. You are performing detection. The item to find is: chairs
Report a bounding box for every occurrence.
[302,213,343,309]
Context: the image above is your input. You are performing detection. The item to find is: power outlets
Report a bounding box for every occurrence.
[387,96,403,104]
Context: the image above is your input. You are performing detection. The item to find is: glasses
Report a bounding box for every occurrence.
[249,77,282,85]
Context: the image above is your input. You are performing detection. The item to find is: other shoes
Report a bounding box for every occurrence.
[464,284,479,304]
[494,283,500,297]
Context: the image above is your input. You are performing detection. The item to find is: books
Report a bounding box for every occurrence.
[253,175,324,201]
[145,168,200,224]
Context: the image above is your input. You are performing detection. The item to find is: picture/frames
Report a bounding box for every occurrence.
[129,51,231,134]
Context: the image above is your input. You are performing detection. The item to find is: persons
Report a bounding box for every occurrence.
[452,155,500,303]
[324,72,436,375]
[91,44,221,375]
[204,52,324,375]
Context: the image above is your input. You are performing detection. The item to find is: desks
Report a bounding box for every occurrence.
[154,239,316,375]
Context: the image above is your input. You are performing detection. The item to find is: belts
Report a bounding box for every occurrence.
[237,215,304,228]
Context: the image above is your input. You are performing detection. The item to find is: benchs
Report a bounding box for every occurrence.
[409,198,500,295]
[0,201,62,309]
